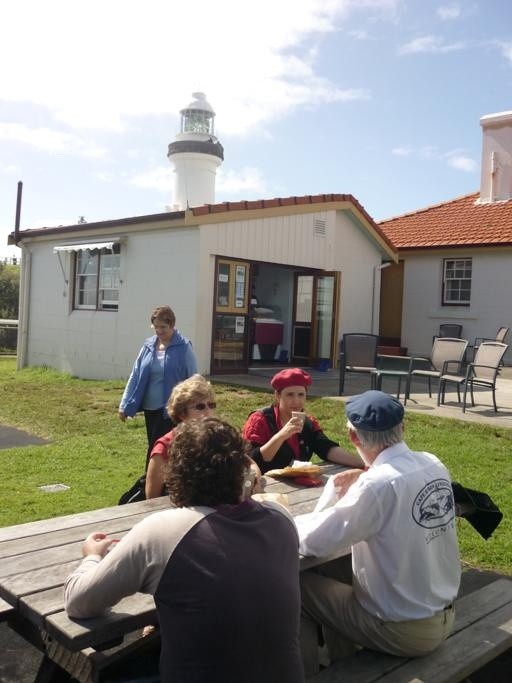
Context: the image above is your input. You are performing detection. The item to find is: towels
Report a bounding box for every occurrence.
[313,475,336,518]
[292,460,312,468]
[290,508,333,544]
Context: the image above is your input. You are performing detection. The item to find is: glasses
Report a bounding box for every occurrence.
[186,401,217,410]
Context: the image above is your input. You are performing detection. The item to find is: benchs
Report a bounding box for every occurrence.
[319,577,512,683]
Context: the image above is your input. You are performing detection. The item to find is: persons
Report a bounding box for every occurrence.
[243,369,364,475]
[118,308,195,469]
[296,390,459,674]
[69,417,300,683]
[147,374,218,498]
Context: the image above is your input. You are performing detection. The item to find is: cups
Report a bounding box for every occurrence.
[291,411,305,432]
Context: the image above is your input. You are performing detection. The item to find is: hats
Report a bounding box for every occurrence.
[345,389,404,431]
[270,368,311,391]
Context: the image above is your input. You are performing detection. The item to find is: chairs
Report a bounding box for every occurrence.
[339,333,384,396]
[404,336,469,406]
[474,327,509,367]
[433,324,463,344]
[437,341,510,413]
